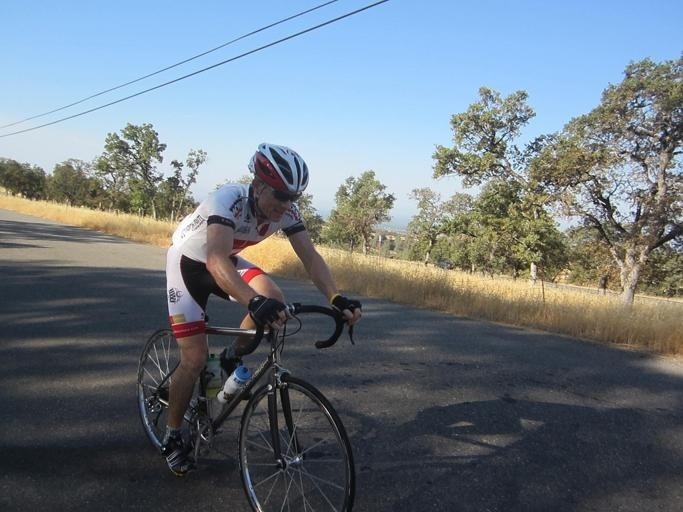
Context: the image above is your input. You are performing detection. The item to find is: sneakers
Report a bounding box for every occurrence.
[220,348,252,400]
[162,438,190,476]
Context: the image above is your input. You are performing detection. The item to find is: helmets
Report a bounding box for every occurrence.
[249,144,308,195]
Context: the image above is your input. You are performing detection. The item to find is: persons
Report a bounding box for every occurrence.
[161,142,362,478]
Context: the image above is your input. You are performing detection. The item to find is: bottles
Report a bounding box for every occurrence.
[216,366,252,407]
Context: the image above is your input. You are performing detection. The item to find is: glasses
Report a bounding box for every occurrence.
[271,189,302,202]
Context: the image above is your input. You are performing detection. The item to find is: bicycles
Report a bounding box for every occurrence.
[136,303,355,512]
